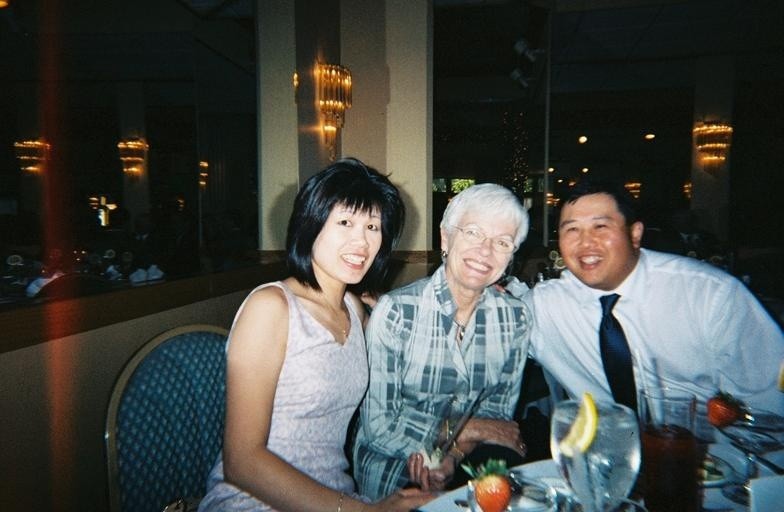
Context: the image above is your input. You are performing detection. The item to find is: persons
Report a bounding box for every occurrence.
[352,180,533,501]
[518,184,784,440]
[198,157,437,512]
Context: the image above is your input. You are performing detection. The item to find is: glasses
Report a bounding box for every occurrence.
[451,225,517,255]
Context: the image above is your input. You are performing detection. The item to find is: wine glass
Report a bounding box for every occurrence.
[716,402,782,505]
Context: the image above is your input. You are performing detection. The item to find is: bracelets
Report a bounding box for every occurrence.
[337,490,345,512]
[445,416,456,441]
[452,446,466,459]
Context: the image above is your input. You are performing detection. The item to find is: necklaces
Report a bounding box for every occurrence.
[320,293,351,342]
[452,304,477,340]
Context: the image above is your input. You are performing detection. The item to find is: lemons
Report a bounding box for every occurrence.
[556,392,597,457]
[776,363,784,391]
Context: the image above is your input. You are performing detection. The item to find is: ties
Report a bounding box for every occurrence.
[596,294,639,420]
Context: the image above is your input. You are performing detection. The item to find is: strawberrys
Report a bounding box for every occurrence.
[706,389,745,428]
[474,457,513,512]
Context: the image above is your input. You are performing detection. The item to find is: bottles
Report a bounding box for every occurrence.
[536,266,561,282]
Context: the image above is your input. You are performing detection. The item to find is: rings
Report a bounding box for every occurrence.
[519,441,526,450]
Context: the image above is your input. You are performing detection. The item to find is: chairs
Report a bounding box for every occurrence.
[106,321,243,511]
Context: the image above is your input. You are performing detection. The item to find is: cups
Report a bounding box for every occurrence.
[547,398,644,511]
[637,389,696,512]
